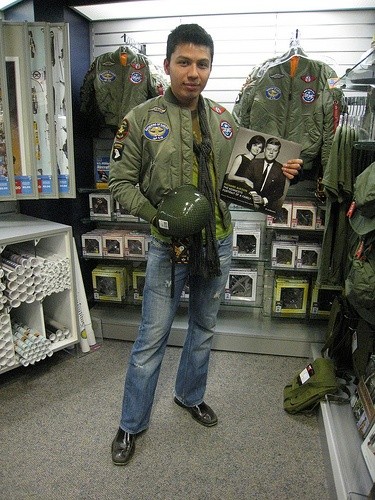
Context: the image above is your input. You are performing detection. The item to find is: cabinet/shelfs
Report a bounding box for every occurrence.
[0,212,79,373]
[78,188,330,358]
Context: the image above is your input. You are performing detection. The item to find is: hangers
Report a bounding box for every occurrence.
[338,96,367,131]
[121,35,141,54]
[280,40,309,63]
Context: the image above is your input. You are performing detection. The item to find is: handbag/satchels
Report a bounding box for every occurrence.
[283,358,338,414]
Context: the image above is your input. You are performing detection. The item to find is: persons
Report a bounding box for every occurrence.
[108,24,305,464]
[221,135,287,211]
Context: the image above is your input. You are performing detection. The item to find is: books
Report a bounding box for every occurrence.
[220,126,302,218]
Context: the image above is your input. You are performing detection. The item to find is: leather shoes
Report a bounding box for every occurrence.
[174,396,218,426]
[111,427,137,465]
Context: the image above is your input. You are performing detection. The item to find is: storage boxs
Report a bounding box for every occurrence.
[82,192,342,319]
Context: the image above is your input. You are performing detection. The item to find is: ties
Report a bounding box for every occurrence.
[259,164,269,190]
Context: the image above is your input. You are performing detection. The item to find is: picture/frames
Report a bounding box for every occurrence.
[50,22,76,198]
[3,21,38,200]
[0,18,16,201]
[29,23,59,199]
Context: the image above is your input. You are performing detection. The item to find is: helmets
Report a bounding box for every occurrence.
[156,184,210,236]
